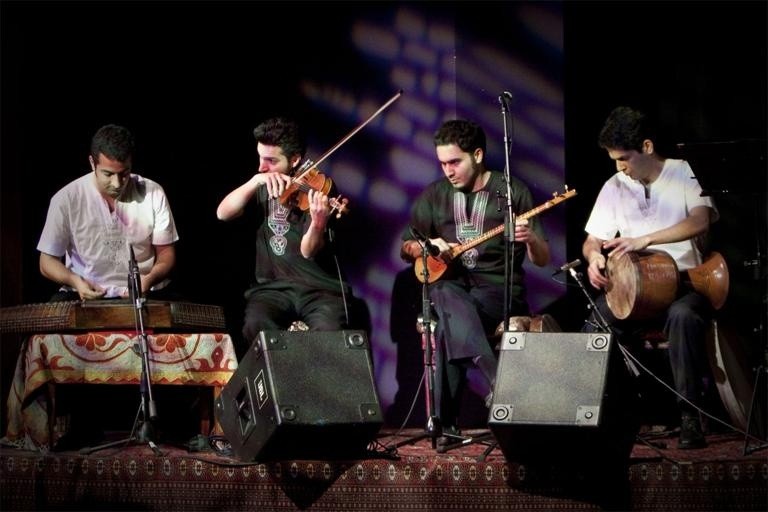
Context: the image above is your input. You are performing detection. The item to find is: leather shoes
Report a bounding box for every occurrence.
[677,415,705,449]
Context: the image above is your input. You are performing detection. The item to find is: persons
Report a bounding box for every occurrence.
[580,106,720,450]
[36,126,181,304]
[400,119,549,445]
[216,120,353,346]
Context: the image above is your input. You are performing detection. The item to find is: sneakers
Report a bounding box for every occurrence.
[181,435,211,452]
[53,430,91,452]
[437,428,461,446]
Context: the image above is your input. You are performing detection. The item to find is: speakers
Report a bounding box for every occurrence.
[487,332,642,461]
[215,330,383,464]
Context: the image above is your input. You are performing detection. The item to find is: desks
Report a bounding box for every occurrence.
[33,332,236,449]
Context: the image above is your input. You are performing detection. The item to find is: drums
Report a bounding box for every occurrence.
[605,249,730,321]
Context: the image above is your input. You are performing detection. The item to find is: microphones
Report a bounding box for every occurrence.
[411,226,440,257]
[551,259,581,277]
[499,90,513,108]
[127,257,142,303]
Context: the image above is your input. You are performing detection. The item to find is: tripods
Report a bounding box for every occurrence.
[435,92,515,461]
[743,363,768,456]
[78,243,195,457]
[386,248,500,454]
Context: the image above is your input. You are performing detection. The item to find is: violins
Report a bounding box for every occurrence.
[281,158,349,220]
